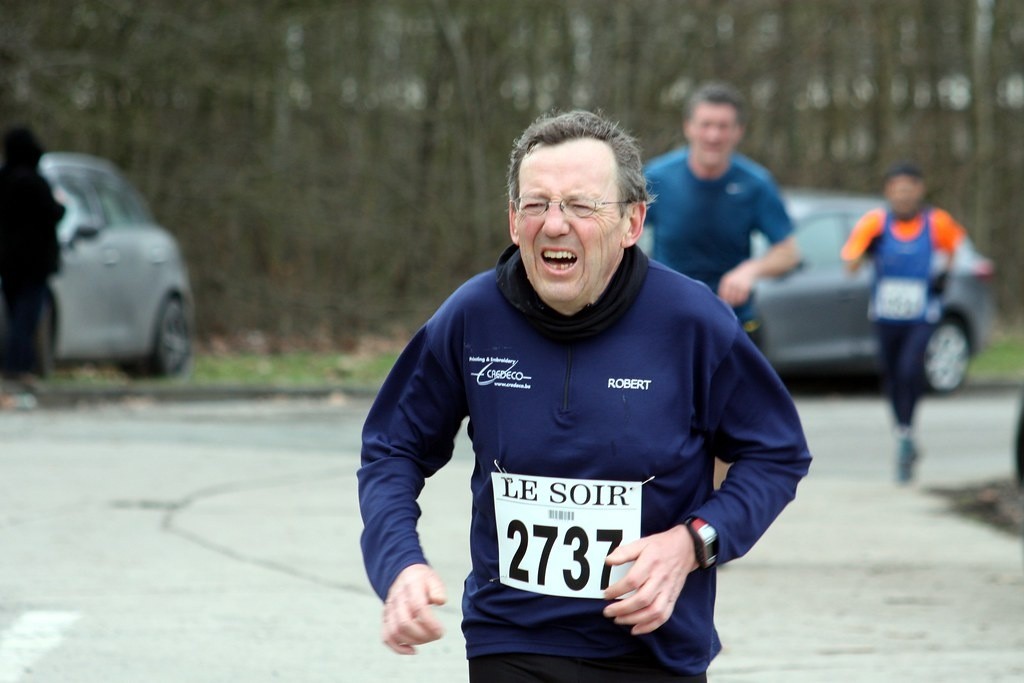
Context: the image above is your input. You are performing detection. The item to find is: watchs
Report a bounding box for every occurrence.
[680,515,720,568]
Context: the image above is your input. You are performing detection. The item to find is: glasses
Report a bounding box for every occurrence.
[511,195,628,217]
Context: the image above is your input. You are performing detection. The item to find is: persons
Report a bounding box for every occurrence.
[353,109,813,683]
[0,127,67,411]
[635,78,804,493]
[836,164,978,488]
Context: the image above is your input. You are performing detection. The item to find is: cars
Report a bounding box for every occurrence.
[30,151,195,382]
[627,189,997,394]
